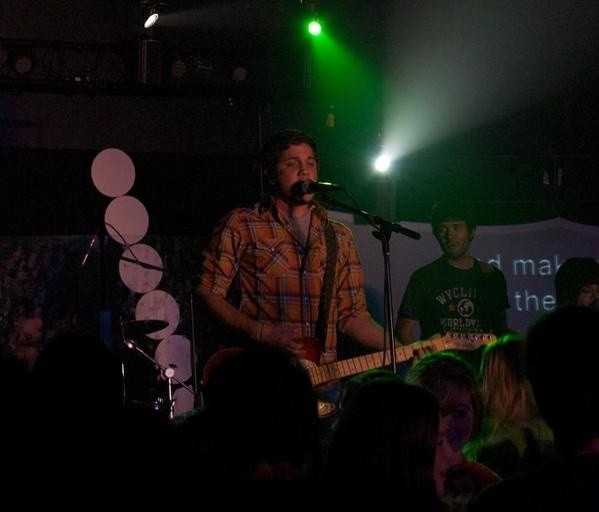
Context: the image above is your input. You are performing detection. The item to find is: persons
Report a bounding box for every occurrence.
[395,196,513,346]
[0,302,599,510]
[194,131,443,438]
[553,254,599,313]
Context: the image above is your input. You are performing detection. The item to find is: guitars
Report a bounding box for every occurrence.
[203,328,497,418]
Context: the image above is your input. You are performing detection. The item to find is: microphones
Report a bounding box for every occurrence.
[77,237,98,268]
[302,177,347,196]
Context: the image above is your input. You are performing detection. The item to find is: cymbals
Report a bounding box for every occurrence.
[122,320,168,336]
